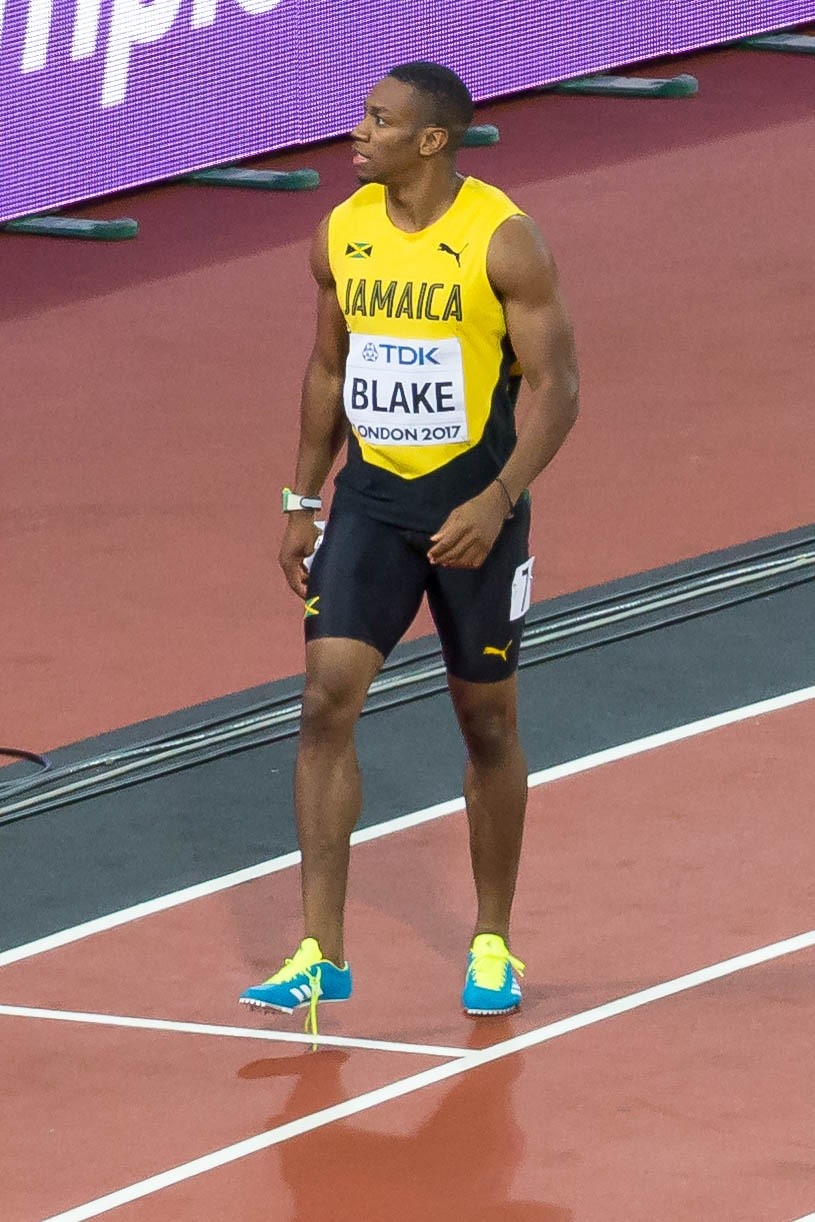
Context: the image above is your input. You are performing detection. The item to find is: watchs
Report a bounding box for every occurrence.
[278,486,323,513]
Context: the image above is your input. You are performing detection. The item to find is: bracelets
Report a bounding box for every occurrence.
[496,477,515,516]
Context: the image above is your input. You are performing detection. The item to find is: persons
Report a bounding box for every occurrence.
[237,56,583,1020]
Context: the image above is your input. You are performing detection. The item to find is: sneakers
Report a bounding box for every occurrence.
[239,939,354,1052]
[462,933,526,1020]
